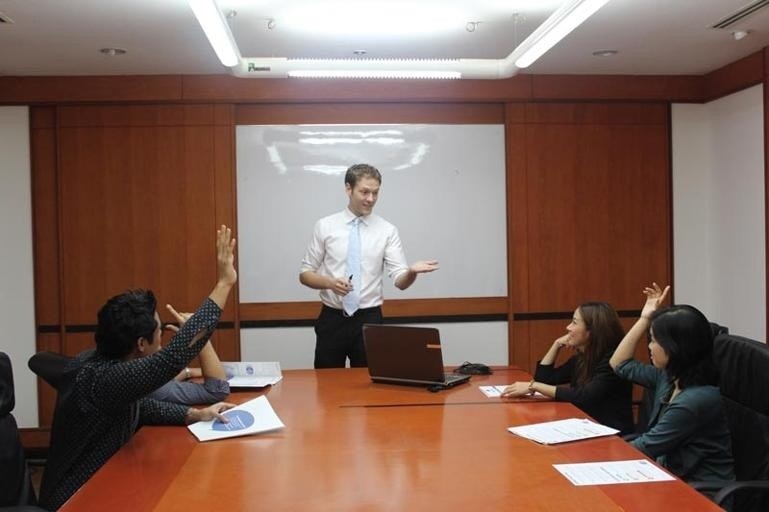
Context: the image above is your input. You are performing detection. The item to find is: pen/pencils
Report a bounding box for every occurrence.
[348,274,354,283]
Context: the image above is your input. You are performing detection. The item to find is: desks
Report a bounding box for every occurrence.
[57,364,726,511]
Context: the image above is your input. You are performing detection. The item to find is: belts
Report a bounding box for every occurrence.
[323,306,381,316]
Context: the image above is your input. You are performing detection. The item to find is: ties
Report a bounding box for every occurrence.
[342,220,361,315]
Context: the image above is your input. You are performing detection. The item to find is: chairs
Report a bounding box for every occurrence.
[29,349,76,391]
[716,480,769,511]
[626,319,730,441]
[651,335,768,511]
[0,352,43,511]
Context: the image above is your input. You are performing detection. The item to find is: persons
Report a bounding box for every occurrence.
[39,224,238,511]
[609,281,737,512]
[299,164,440,369]
[498,302,635,438]
[144,303,231,405]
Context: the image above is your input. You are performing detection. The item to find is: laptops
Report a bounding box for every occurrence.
[361,323,472,392]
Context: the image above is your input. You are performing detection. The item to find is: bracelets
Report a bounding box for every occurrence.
[528,380,535,396]
[185,368,192,379]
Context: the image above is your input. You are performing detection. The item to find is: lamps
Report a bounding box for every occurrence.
[187,0,241,67]
[514,0,609,69]
[287,68,462,80]
